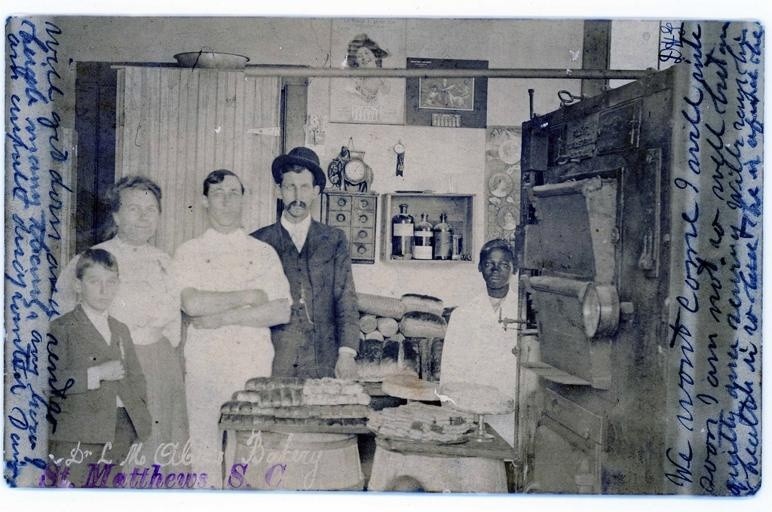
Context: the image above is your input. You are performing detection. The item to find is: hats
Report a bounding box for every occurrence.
[271,146,326,194]
[480,238,516,256]
[347,34,389,67]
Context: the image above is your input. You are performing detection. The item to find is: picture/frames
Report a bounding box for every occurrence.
[404,57,489,129]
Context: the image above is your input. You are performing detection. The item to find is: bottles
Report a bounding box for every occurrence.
[392,203,453,260]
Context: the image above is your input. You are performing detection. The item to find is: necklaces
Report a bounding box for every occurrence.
[356,81,383,106]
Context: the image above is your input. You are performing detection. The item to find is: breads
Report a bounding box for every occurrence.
[221,294,514,442]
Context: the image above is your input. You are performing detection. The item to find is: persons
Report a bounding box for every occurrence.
[440,238,533,448]
[46,146,360,488]
[344,34,390,106]
